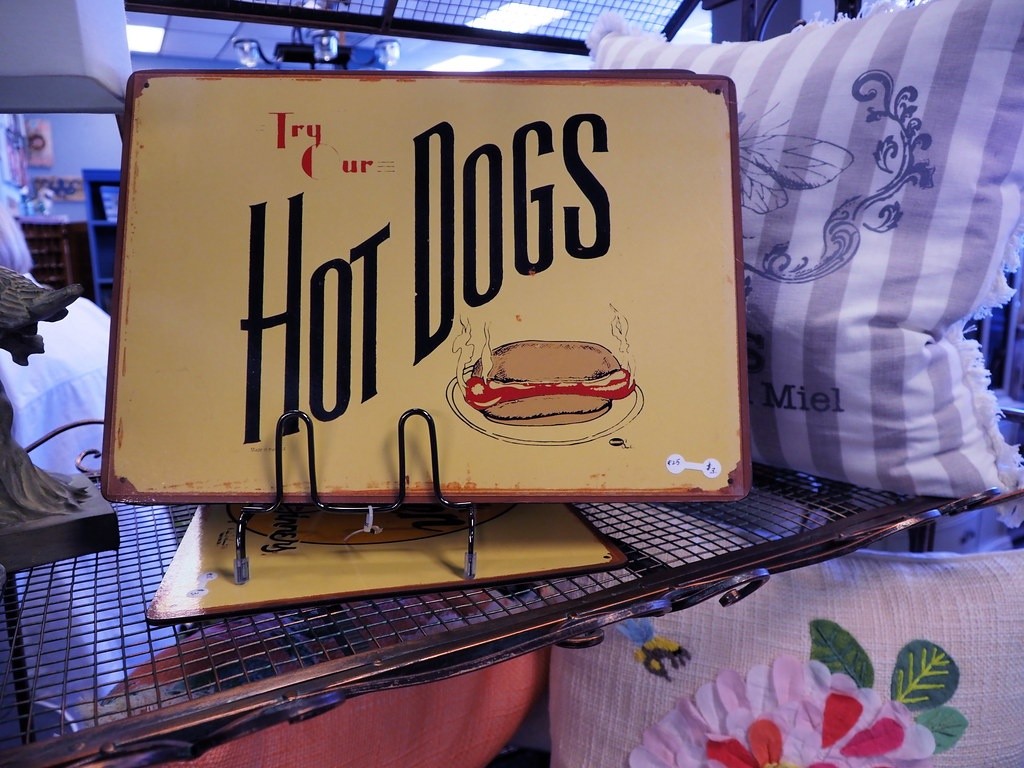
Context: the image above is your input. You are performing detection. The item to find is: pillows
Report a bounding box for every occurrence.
[547,537,1024,768]
[69,566,557,768]
[583,0,1024,500]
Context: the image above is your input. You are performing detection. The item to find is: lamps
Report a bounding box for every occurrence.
[232,0,401,71]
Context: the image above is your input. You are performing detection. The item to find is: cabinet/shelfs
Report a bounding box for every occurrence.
[21,167,122,309]
